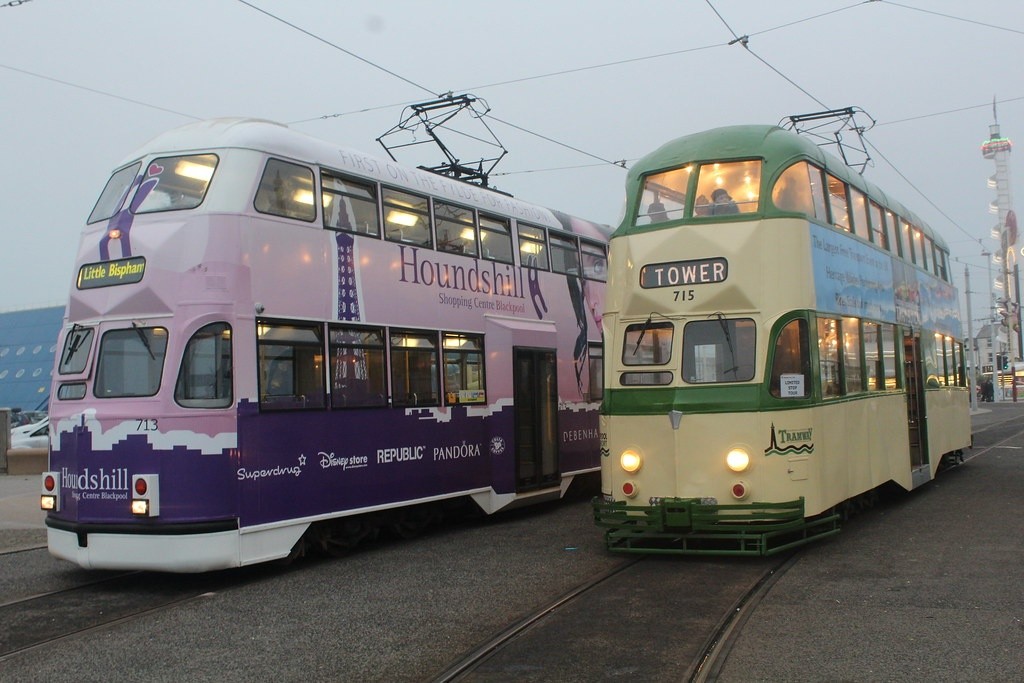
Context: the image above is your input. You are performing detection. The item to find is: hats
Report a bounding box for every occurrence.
[711,189,732,201]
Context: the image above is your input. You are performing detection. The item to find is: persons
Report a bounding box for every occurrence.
[981,379,994,402]
[648,203,670,223]
[552,211,614,386]
[709,190,739,215]
[695,196,711,216]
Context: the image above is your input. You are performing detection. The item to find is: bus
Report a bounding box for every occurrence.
[39,92,672,575]
[588,104,977,559]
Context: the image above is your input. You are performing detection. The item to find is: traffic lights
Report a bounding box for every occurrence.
[1001,354,1008,370]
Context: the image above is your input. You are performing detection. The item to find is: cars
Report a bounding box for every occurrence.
[11,412,49,450]
